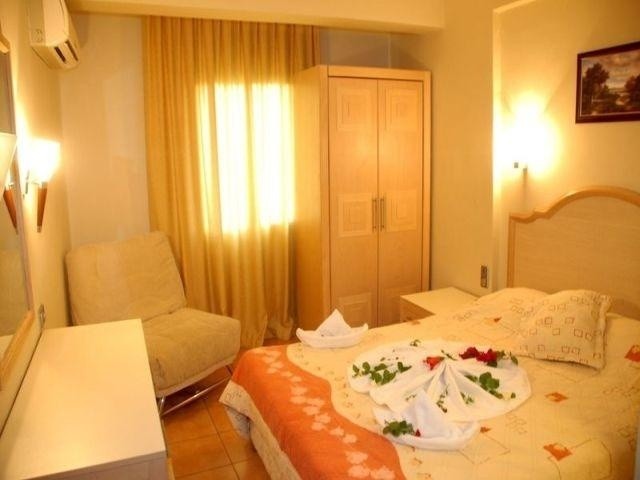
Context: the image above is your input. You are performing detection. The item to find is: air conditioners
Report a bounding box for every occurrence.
[28,0,80,70]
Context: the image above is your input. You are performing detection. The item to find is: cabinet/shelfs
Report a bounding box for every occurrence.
[294,64,433,329]
[5,319,174,479]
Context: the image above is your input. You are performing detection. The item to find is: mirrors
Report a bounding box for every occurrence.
[1,34,42,437]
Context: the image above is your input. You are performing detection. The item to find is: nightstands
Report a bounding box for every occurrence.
[400,287,480,322]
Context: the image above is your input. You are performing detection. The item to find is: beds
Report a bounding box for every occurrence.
[218,184,640,479]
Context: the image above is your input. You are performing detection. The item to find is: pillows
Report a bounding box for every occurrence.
[444,287,548,331]
[510,289,612,369]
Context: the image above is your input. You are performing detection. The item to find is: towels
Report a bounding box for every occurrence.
[296,309,532,451]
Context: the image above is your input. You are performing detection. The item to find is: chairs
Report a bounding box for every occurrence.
[65,231,243,418]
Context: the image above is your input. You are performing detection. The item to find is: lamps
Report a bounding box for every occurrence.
[1,131,19,235]
[23,138,61,233]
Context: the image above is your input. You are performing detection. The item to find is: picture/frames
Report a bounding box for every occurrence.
[575,40,639,122]
